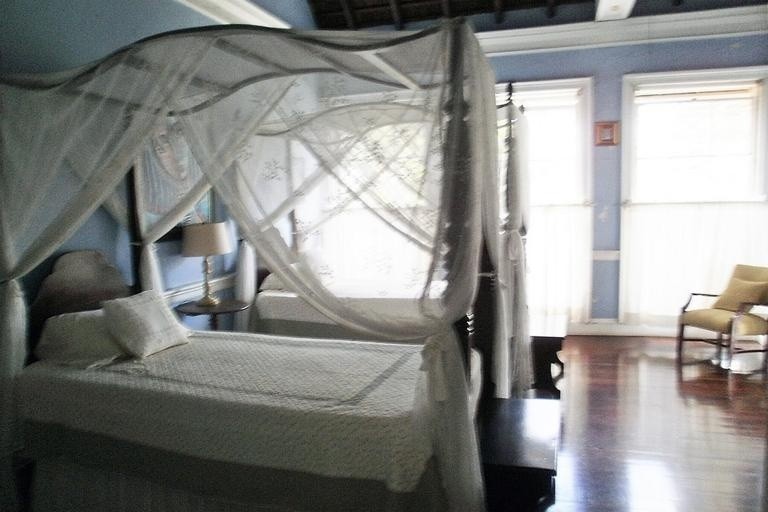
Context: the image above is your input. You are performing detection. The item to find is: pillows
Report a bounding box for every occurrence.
[258,261,320,292]
[98,285,189,358]
[37,307,192,371]
[711,278,768,313]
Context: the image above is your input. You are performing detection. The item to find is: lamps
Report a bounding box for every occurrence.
[177,219,232,307]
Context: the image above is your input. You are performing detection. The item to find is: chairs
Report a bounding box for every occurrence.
[675,263,768,370]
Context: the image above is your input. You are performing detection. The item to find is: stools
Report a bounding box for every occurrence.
[475,393,563,509]
[529,304,568,398]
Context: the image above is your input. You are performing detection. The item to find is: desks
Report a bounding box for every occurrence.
[172,300,254,331]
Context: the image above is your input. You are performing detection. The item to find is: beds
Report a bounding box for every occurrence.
[3,14,500,507]
[230,79,528,401]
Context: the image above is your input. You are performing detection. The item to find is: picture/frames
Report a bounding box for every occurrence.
[593,120,619,146]
